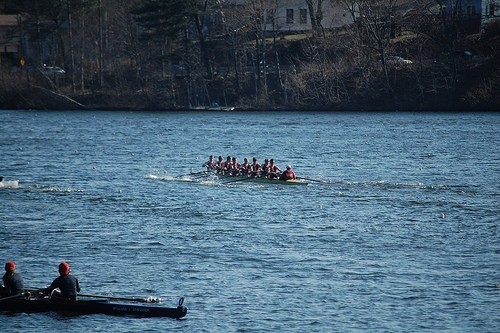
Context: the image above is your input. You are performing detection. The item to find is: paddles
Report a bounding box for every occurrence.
[77,294,163,303]
[277,173,320,182]
[0,289,43,301]
[172,168,248,184]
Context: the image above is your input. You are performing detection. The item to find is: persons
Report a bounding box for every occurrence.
[203,155,283,180]
[44,262,81,302]
[0,262,24,297]
[279,165,296,180]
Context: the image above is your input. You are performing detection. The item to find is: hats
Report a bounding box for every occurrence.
[5,262,15,269]
[59,262,71,275]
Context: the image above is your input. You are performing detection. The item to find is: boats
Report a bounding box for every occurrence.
[213,169,308,186]
[0,287,187,318]
